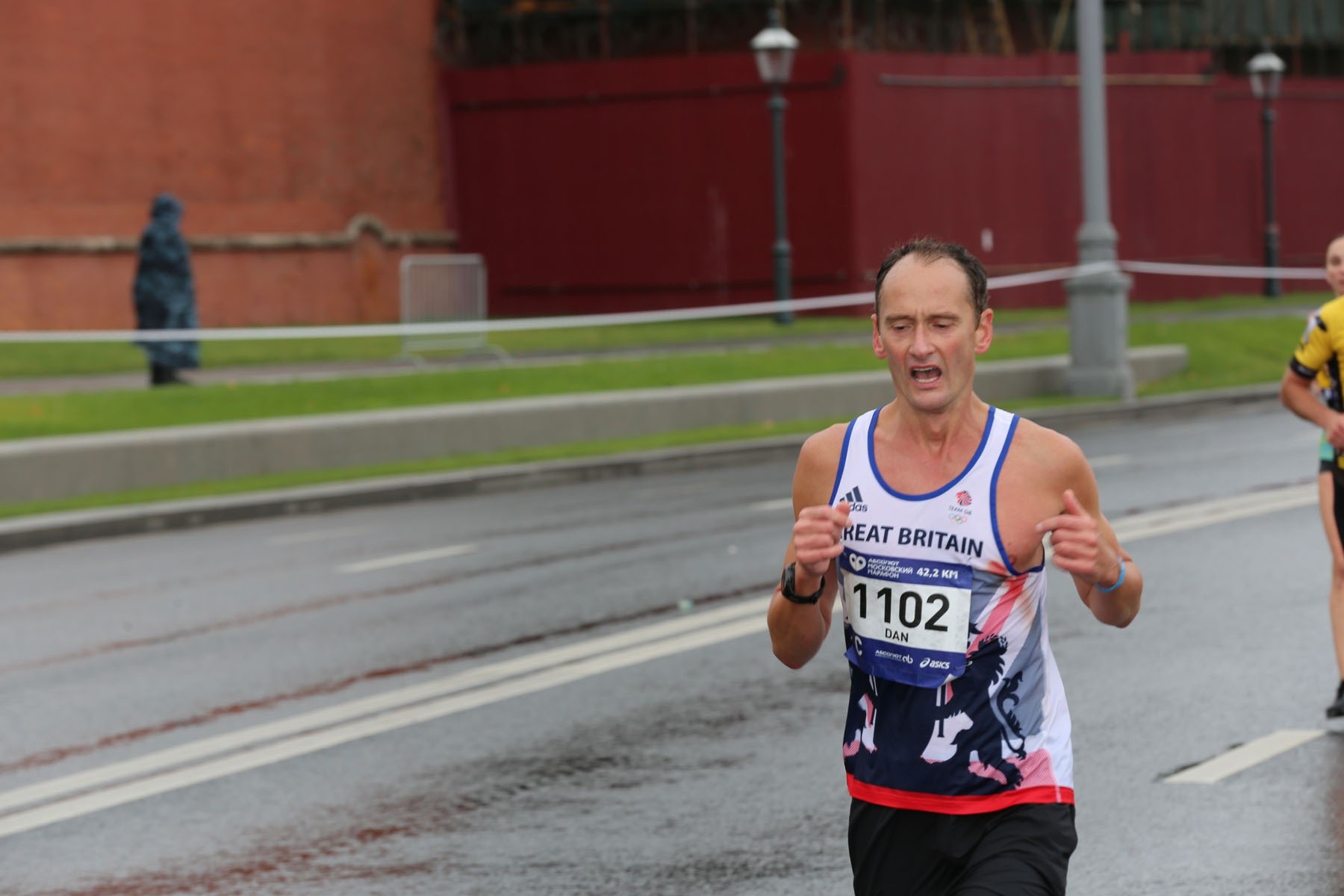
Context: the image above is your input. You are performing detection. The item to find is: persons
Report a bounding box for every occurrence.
[131,191,198,386]
[1279,237,1344,721]
[768,239,1144,896]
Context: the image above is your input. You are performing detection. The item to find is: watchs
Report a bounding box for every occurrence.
[780,561,827,605]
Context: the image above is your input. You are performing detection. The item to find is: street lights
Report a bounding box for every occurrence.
[750,26,796,324]
[1246,52,1286,295]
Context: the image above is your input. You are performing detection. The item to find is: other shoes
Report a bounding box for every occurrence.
[1327,677,1344,732]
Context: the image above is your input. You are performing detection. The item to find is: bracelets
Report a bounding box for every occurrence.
[1094,556,1126,594]
